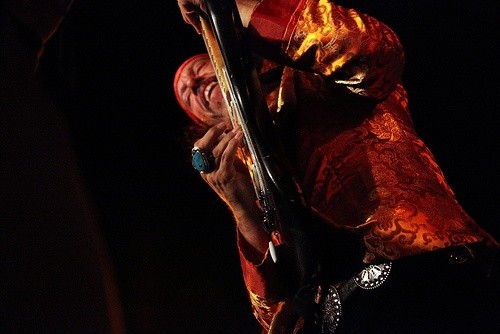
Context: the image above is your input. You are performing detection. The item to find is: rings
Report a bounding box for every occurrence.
[191,146,221,174]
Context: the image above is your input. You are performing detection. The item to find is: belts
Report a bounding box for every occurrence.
[322,260,392,334]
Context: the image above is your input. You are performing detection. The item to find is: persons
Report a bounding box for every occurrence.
[173,0,500,334]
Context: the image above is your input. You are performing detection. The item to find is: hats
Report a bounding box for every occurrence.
[173,54,211,129]
